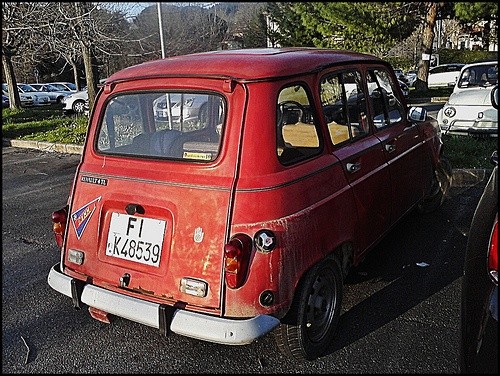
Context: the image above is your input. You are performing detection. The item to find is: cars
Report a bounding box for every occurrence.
[45,46,452,361]
[153,94,224,125]
[2,78,124,114]
[338,64,469,107]
[436,62,498,139]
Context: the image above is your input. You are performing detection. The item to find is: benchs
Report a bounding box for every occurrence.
[282,120,359,147]
